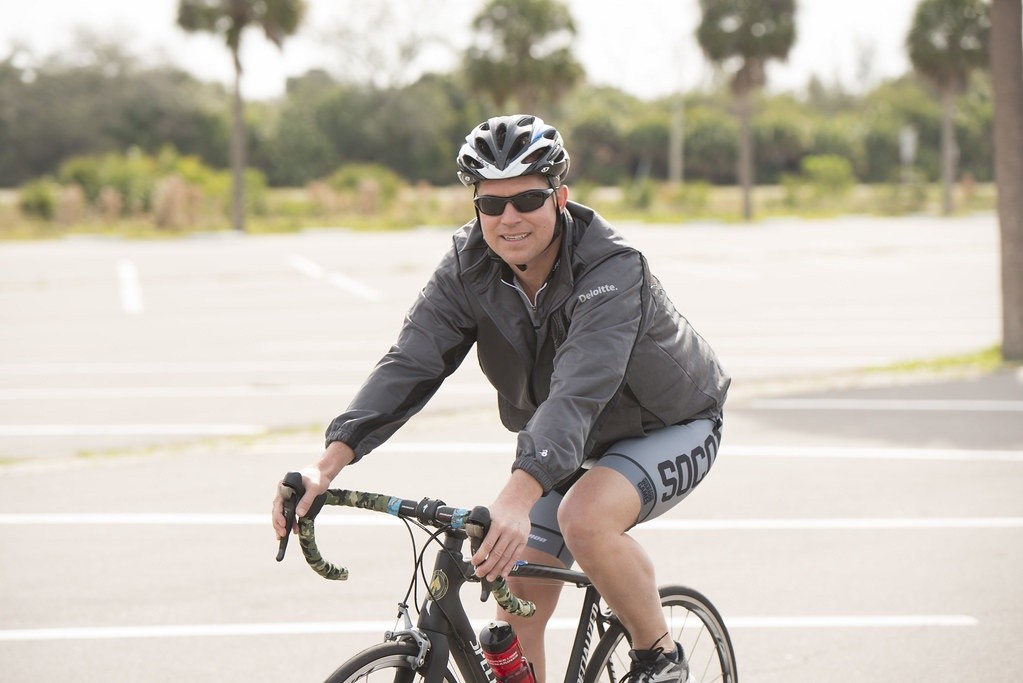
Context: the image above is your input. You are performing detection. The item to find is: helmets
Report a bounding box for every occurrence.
[456,113,571,184]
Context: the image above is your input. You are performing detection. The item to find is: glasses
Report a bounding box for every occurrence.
[472,186,560,216]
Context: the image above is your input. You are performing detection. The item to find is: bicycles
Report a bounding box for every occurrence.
[274,469,738,683]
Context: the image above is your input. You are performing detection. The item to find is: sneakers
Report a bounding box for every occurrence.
[619,630,690,683]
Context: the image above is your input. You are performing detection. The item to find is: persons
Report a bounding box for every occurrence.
[272,115,732,683]
